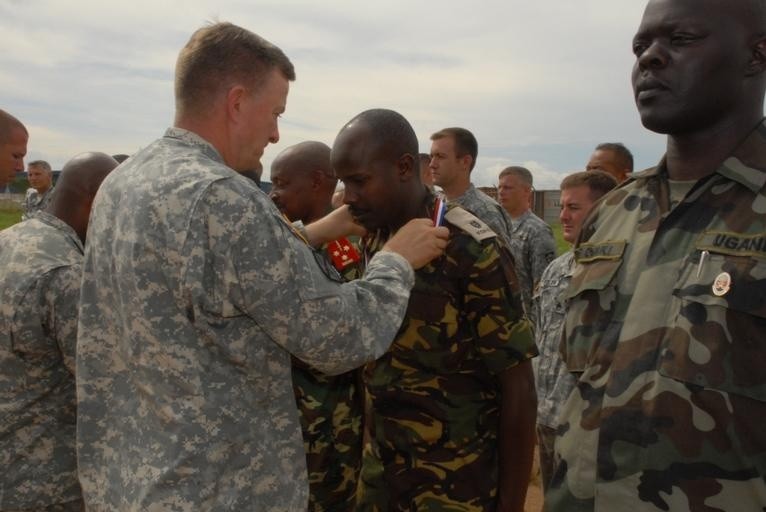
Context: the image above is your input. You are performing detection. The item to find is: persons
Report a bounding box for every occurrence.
[0,152,118,511]
[429,128,514,255]
[531,171,617,512]
[542,0,766,512]
[20,160,54,221]
[498,167,556,333]
[0,108,28,187]
[586,143,633,185]
[76,23,449,512]
[267,142,364,511]
[330,109,540,512]
[418,154,435,194]
